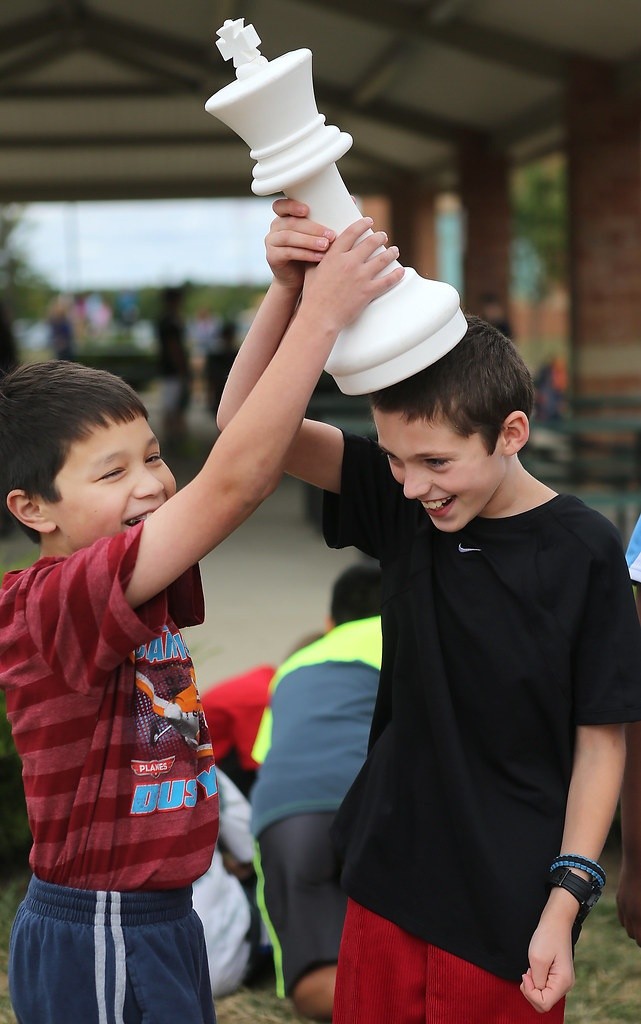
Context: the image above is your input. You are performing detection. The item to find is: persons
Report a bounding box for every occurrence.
[248,561,385,1023]
[194,765,263,999]
[1,216,407,1024]
[199,632,326,804]
[615,511,641,951]
[45,286,240,464]
[216,197,641,1024]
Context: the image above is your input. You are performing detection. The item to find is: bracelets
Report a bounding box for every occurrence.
[549,854,606,889]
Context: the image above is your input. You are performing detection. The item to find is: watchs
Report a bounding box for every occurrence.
[548,866,603,924]
